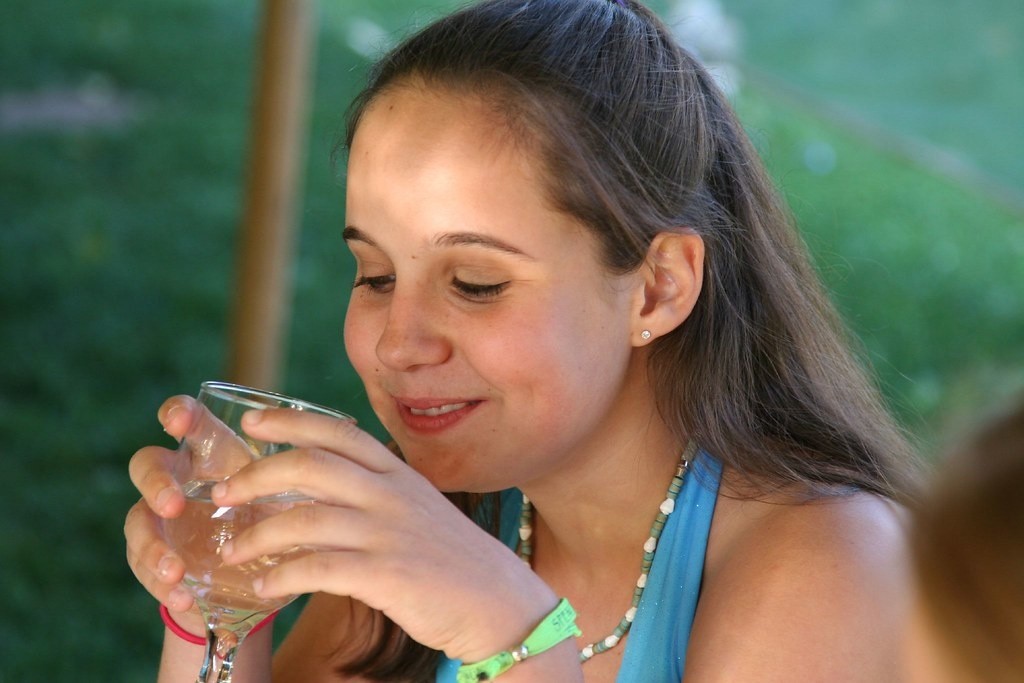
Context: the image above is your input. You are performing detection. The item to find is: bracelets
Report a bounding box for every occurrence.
[458,598,583,683]
[159,605,278,647]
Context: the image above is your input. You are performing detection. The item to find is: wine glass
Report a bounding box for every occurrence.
[160,380,357,683]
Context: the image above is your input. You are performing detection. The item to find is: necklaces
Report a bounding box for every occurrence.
[517,440,694,662]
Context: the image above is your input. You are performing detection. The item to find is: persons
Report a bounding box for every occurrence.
[123,0,934,683]
[916,400,1024,683]
